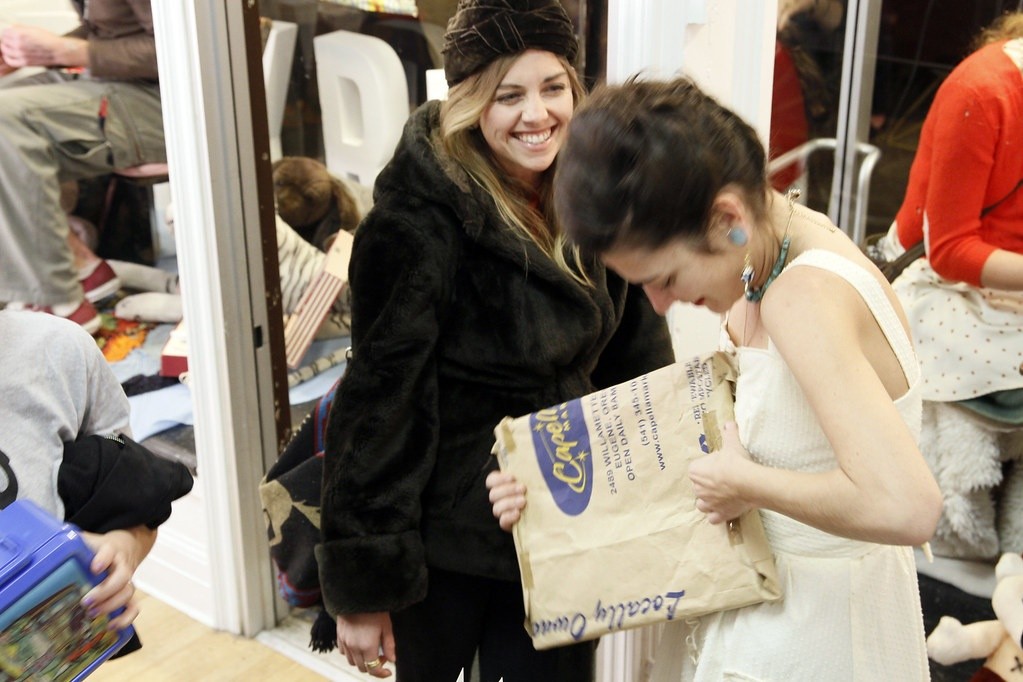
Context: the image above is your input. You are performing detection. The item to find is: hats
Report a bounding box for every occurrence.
[441,0,578,88]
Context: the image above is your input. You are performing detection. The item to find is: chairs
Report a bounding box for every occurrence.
[97,16,304,271]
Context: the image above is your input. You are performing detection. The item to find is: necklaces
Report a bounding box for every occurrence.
[746,188,834,303]
[744,301,761,347]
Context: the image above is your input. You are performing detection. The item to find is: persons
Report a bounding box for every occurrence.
[771,42,809,206]
[485,79,943,682]
[320,0,673,682]
[876,9,1023,399]
[0,1,167,629]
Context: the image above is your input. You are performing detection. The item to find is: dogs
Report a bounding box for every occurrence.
[913,385,1023,563]
[271,159,374,252]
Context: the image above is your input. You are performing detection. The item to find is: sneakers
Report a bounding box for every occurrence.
[33,299,102,335]
[24,260,120,308]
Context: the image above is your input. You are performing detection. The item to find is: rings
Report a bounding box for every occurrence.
[365,659,380,668]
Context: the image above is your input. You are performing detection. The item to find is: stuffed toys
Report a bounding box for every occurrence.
[109,204,353,342]
[920,399,1023,562]
[926,552,1023,682]
[272,156,361,249]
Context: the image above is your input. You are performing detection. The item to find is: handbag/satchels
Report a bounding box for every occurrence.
[859,233,895,285]
[258,376,342,655]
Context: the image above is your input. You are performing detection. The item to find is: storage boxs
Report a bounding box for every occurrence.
[492,344,788,652]
